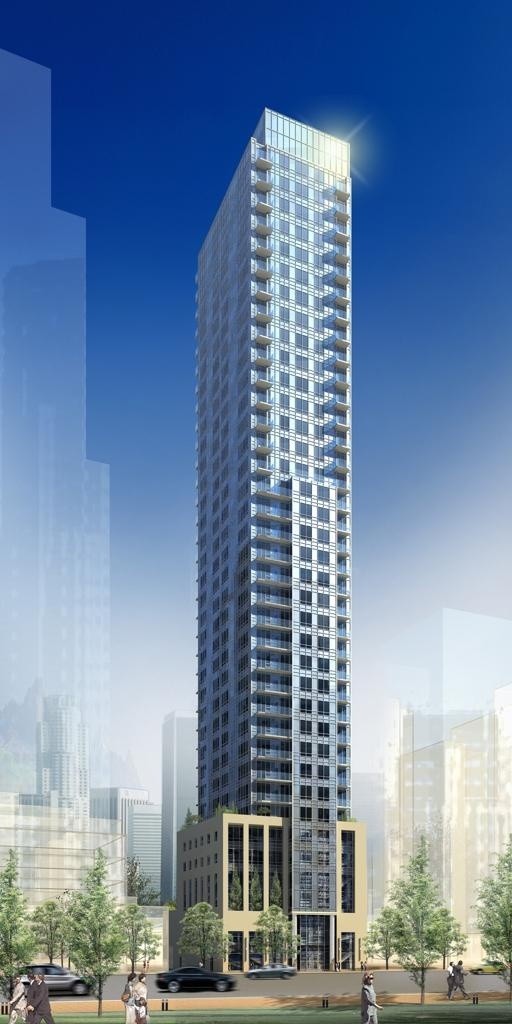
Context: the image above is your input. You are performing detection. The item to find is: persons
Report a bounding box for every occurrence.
[445,962,455,1000]
[335,962,340,972]
[361,971,384,1024]
[21,972,38,1024]
[136,973,150,1023]
[134,997,148,1024]
[450,960,472,1000]
[360,961,364,972]
[7,975,27,1024]
[122,972,138,1024]
[199,960,204,968]
[364,962,368,971]
[27,974,56,1024]
